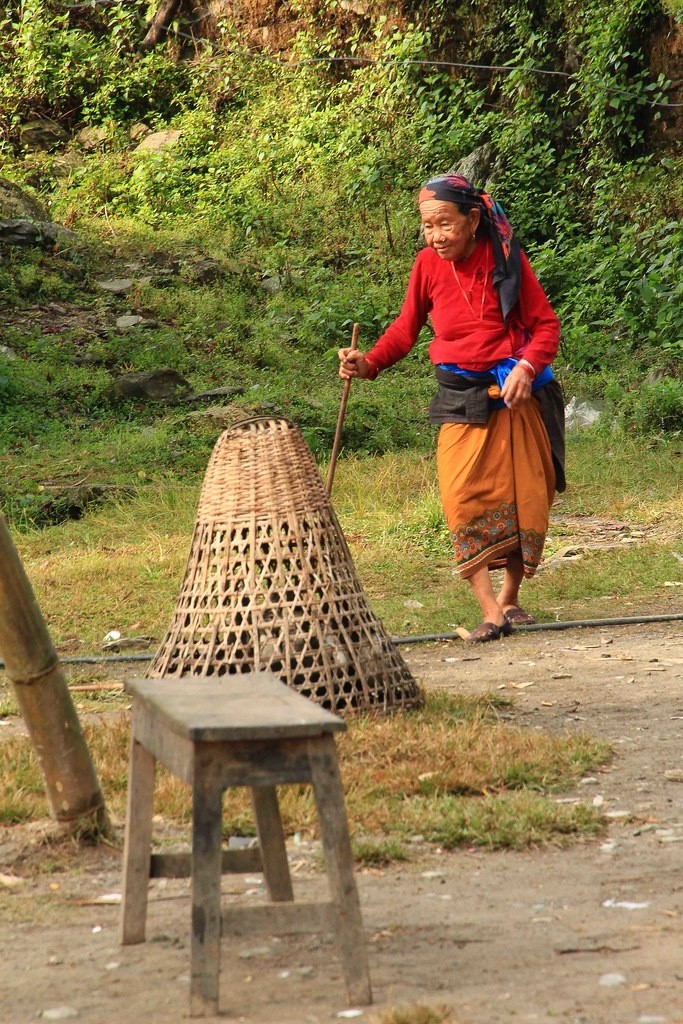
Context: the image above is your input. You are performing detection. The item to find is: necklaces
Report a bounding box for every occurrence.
[450,240,489,324]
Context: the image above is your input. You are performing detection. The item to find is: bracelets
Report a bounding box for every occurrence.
[517,362,535,377]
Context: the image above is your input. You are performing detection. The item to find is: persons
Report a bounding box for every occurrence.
[339,174,566,641]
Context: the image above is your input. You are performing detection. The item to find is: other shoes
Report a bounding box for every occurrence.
[465,614,512,641]
[503,605,536,624]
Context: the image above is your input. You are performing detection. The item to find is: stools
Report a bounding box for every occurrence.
[118,669,373,1019]
